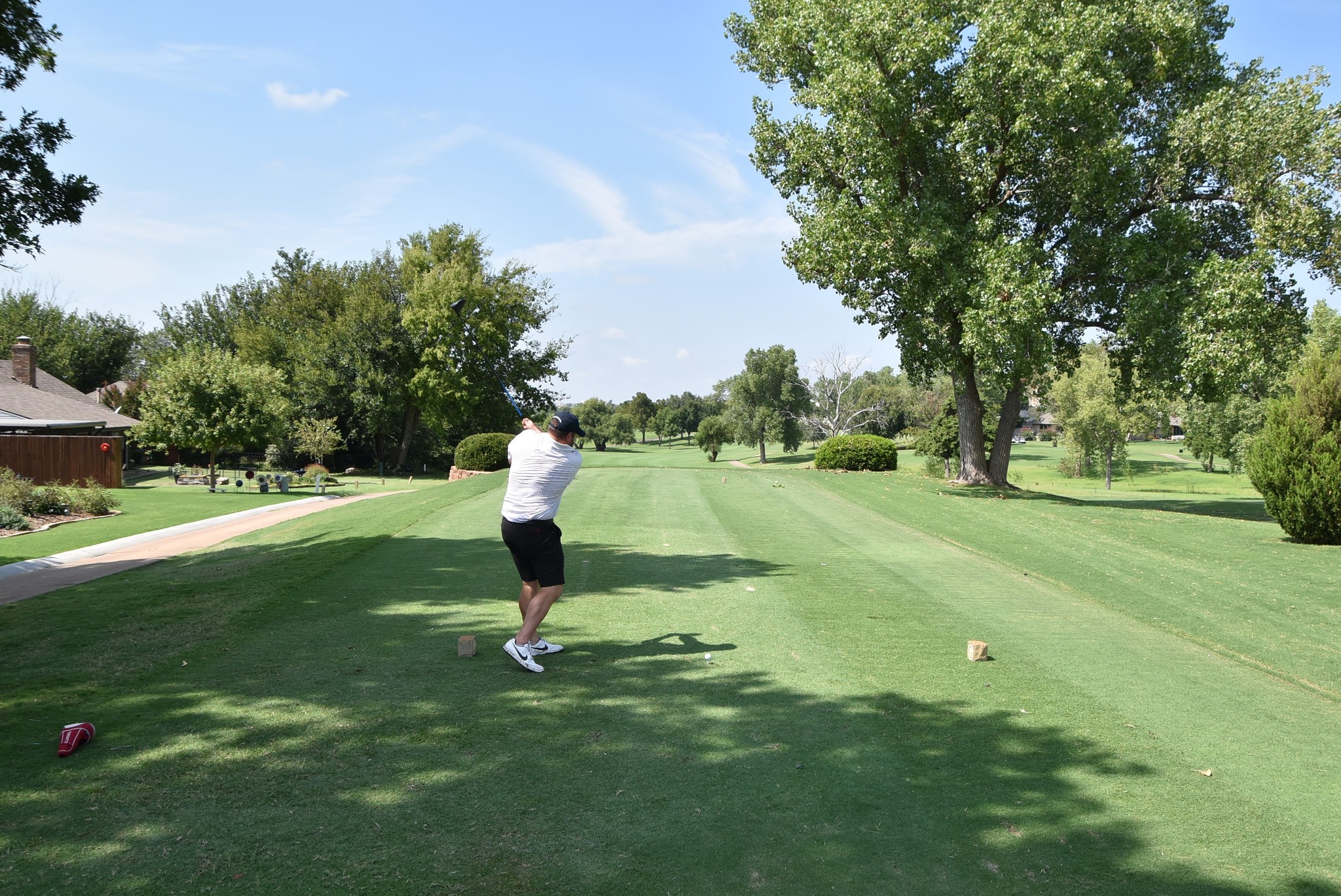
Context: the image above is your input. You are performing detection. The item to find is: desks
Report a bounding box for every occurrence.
[1014,435,1025,438]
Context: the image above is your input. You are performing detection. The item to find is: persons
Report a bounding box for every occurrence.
[501,412,583,672]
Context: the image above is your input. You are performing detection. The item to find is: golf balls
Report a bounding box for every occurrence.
[705,654,712,660]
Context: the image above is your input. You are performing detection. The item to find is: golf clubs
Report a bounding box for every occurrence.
[449,298,525,418]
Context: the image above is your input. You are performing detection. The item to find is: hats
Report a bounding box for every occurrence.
[548,411,586,438]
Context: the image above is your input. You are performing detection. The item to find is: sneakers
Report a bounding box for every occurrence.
[502,637,545,673]
[530,636,564,656]
